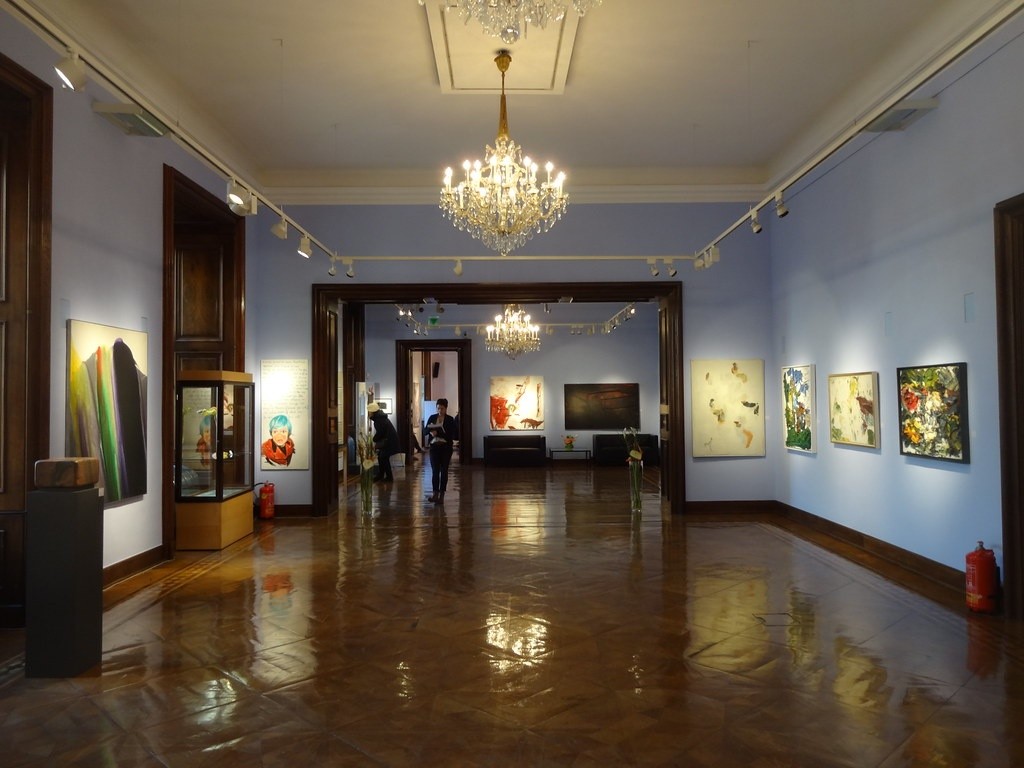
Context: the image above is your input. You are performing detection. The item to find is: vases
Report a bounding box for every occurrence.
[361,464,372,516]
[629,461,642,512]
[565,444,573,450]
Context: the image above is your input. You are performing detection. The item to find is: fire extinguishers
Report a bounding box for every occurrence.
[254,480,275,518]
[965,541,996,613]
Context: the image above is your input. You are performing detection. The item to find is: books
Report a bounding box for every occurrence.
[427,424,445,433]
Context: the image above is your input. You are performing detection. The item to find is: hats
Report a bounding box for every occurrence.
[367,403,380,411]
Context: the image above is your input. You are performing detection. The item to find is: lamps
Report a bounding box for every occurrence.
[54,47,88,91]
[227,0,787,357]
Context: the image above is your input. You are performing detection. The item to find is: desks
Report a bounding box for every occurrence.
[549,448,591,467]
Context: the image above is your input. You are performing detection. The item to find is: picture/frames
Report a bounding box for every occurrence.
[781,364,816,454]
[897,362,971,465]
[828,372,881,448]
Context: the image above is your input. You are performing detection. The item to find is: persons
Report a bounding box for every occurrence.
[367,403,402,482]
[413,435,425,453]
[423,398,459,503]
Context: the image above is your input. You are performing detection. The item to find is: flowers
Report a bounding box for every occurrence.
[561,434,578,445]
[622,427,643,507]
[357,428,379,510]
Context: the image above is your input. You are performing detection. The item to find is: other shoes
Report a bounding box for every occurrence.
[428,491,439,502]
[417,448,427,453]
[434,497,444,504]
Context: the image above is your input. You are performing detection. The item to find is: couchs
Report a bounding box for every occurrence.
[593,434,659,468]
[483,435,547,467]
